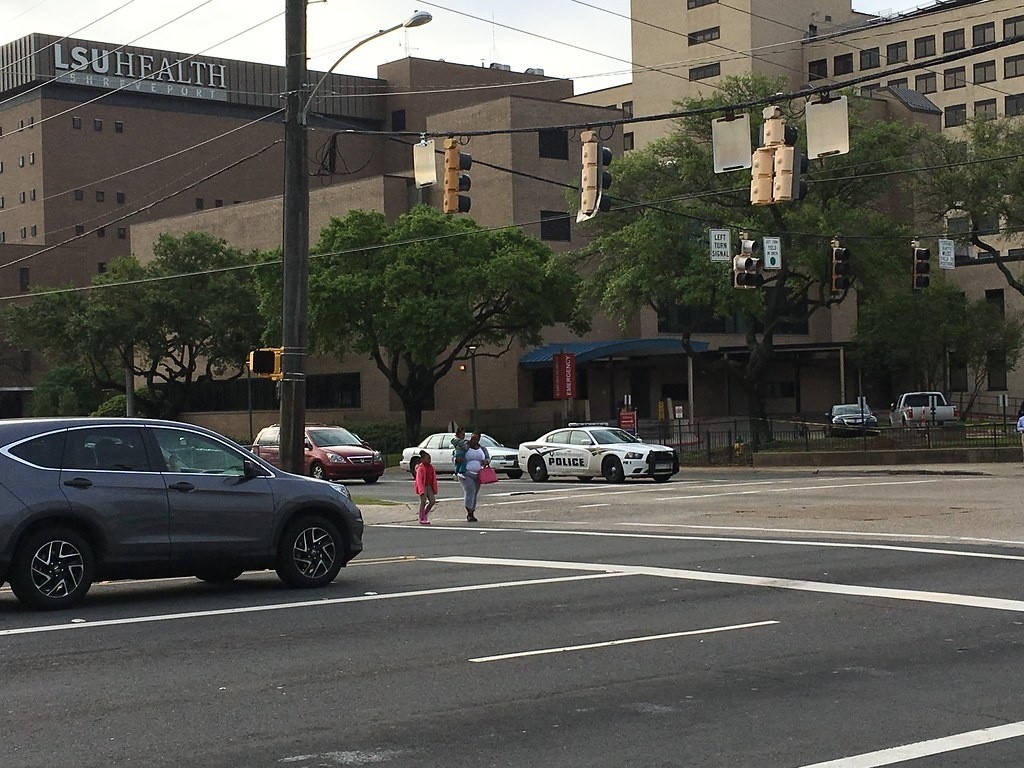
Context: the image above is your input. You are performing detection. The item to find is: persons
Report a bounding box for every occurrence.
[452,430,491,522]
[450,429,471,478]
[414,452,438,524]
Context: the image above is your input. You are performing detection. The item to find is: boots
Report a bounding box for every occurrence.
[419,505,431,524]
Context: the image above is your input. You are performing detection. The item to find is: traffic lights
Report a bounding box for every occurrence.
[442,148,473,212]
[581,143,613,214]
[763,119,798,146]
[249,347,280,378]
[912,247,930,290]
[733,254,764,289]
[831,247,851,292]
[741,239,761,256]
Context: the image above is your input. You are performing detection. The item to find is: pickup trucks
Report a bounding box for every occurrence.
[888,391,958,428]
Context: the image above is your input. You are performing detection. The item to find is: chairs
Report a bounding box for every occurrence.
[71,445,148,471]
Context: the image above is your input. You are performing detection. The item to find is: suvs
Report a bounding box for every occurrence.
[249,420,384,484]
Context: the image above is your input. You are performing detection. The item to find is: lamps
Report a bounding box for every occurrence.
[460,365,467,372]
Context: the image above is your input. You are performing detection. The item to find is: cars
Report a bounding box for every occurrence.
[515,420,681,485]
[0,417,367,613]
[397,431,524,480]
[824,402,880,438]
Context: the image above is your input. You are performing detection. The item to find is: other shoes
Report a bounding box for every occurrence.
[457,473,466,478]
[453,474,457,478]
[467,515,477,522]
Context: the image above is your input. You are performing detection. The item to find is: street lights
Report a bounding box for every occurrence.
[278,9,435,477]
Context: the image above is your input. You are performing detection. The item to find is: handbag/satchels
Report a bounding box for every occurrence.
[478,463,498,484]
